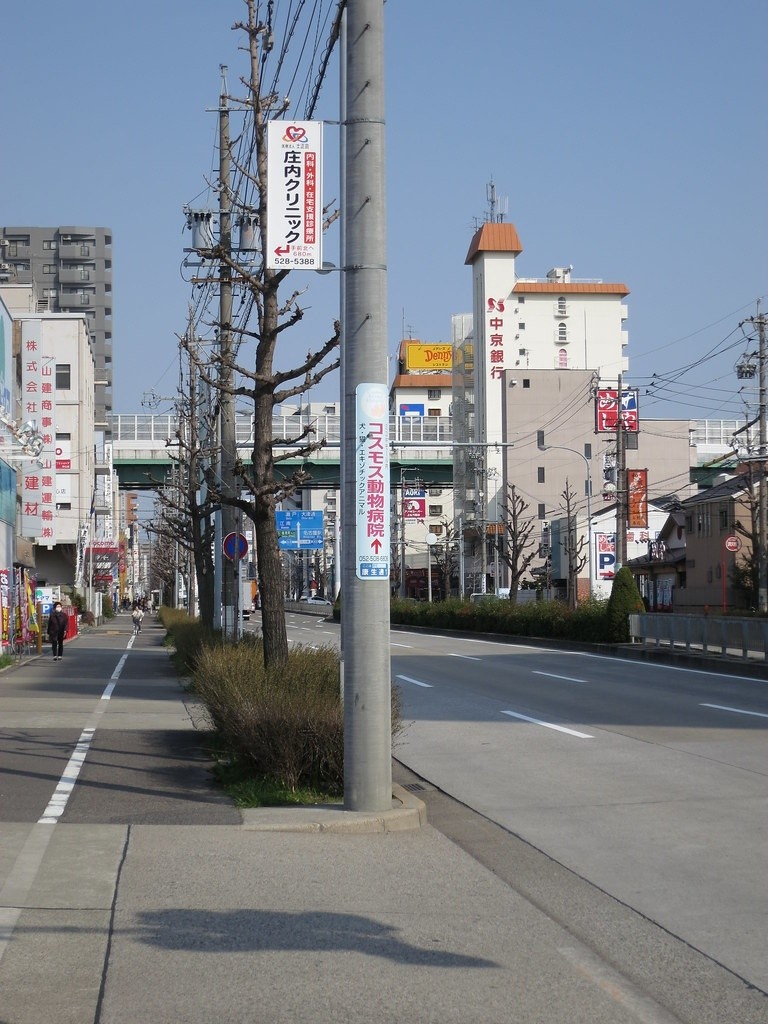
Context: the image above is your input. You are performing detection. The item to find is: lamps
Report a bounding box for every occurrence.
[9,419,36,438]
[21,434,46,456]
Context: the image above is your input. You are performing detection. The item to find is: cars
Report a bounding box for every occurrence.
[470,592,501,608]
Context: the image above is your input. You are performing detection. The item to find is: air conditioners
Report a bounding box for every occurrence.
[0,239,9,246]
[60,309,69,312]
[63,235,71,240]
[1,264,9,269]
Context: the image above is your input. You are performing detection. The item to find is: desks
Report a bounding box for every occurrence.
[62,606,77,639]
[42,614,50,640]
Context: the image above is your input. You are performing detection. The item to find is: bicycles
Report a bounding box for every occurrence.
[131,614,142,636]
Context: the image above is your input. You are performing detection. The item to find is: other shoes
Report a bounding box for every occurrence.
[53,655,57,660]
[58,656,62,660]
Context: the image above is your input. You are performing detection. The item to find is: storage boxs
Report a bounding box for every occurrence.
[36,586,60,614]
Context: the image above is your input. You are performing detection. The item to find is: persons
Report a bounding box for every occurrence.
[47,601,68,662]
[147,599,153,615]
[131,606,145,633]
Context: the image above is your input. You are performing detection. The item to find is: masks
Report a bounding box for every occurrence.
[55,607,61,612]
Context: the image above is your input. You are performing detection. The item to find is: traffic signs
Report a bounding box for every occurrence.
[274,508,326,552]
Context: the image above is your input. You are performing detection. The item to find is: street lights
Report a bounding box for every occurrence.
[537,444,593,607]
